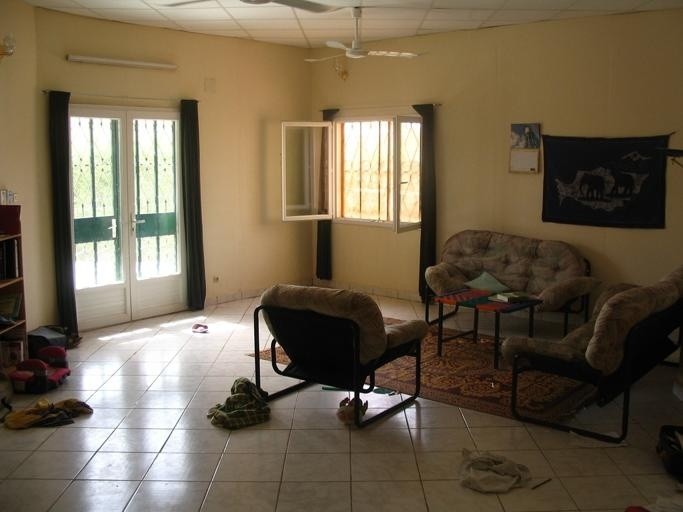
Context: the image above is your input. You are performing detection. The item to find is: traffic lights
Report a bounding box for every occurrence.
[465,272,508,291]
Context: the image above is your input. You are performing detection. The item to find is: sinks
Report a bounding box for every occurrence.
[425,230,591,339]
[502,268,683,444]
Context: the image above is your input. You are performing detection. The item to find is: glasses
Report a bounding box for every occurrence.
[0,32,17,59]
[67,55,178,70]
[334,61,348,80]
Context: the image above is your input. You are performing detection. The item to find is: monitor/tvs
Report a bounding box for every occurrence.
[0,239,20,279]
[487,290,541,303]
[0,339,25,365]
[0,293,22,319]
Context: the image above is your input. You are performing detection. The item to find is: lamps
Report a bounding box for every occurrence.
[254,282,426,428]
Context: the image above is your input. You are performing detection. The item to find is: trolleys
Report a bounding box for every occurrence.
[166,0,344,14]
[305,8,425,61]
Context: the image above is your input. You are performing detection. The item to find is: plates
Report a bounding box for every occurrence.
[0,204,28,412]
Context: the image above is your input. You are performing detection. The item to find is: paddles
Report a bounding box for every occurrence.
[248,316,590,426]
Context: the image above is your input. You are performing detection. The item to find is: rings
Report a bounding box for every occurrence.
[532,478,552,490]
